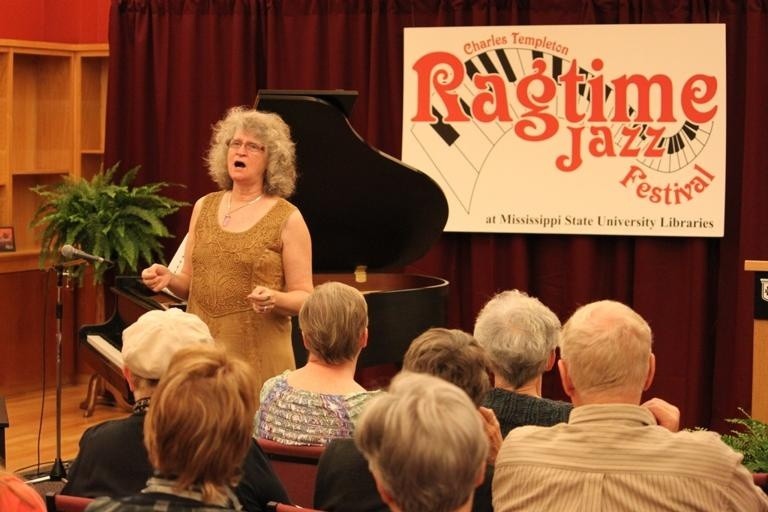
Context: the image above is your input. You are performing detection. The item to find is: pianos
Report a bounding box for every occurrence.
[78,89,450,406]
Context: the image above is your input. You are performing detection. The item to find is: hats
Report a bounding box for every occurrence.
[122,307,215,379]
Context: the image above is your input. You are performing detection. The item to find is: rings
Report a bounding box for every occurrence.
[265,306,268,311]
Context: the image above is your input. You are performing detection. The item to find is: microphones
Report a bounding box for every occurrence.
[59,244,113,266]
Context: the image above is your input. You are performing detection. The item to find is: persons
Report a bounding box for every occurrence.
[1,468,46,512]
[141,104,313,381]
[312,289,768,510]
[254,281,386,447]
[60,307,294,511]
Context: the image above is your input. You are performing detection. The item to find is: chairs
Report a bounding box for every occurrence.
[45,492,95,511]
[254,437,326,508]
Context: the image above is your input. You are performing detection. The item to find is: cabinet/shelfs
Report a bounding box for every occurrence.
[1,38,109,276]
[1,262,111,398]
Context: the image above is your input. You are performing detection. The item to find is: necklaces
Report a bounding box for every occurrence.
[222,190,266,228]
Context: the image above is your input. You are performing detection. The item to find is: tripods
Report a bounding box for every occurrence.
[23,258,84,484]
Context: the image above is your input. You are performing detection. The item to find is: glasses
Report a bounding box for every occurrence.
[229,140,259,151]
